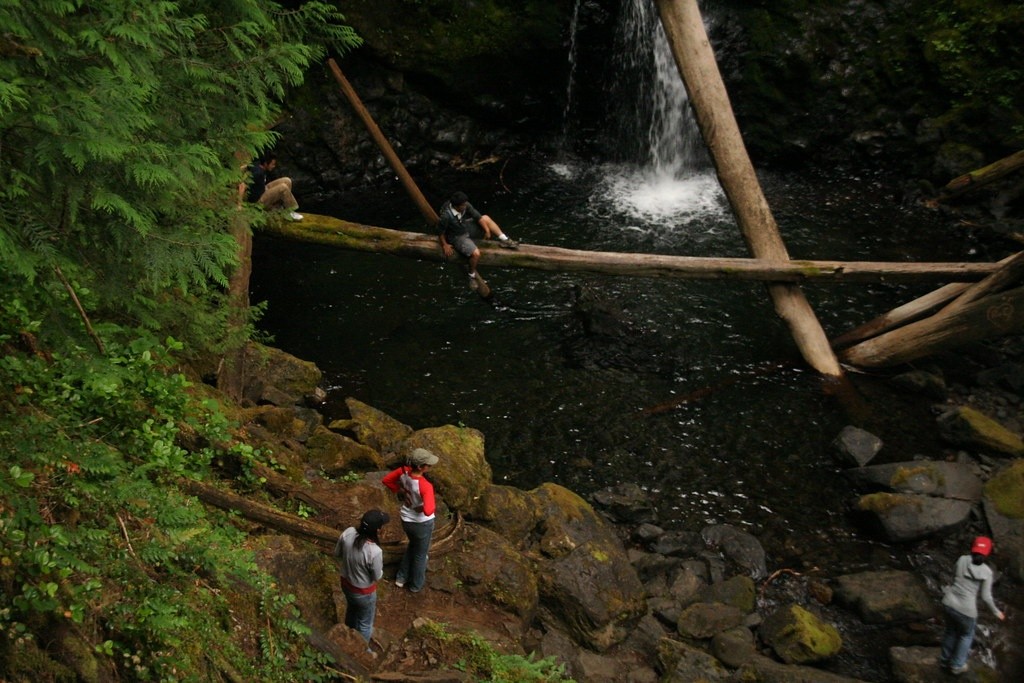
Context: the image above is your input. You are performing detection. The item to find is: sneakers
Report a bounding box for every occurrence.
[284,211,303,221]
[466,274,479,290]
[499,237,520,248]
[941,660,969,674]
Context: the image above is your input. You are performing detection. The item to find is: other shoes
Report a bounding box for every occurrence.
[411,585,421,592]
[395,577,405,587]
[368,647,378,659]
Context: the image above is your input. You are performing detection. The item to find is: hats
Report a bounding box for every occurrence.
[971,535,992,557]
[410,448,439,465]
[362,510,389,531]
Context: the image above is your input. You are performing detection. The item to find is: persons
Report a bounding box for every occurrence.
[238,151,304,221]
[437,192,522,288]
[333,510,391,658]
[381,448,440,592]
[938,536,1005,675]
